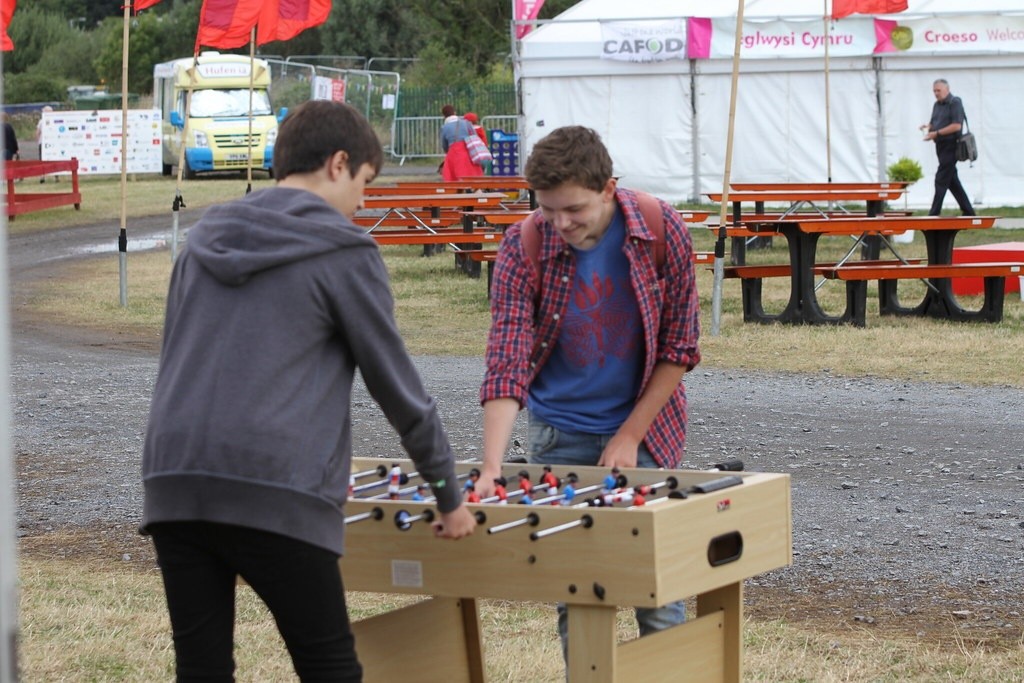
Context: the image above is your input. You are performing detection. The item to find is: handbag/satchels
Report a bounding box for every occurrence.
[465,122,492,166]
[955,114,978,162]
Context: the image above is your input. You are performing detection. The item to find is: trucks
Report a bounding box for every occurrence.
[151,51,287,179]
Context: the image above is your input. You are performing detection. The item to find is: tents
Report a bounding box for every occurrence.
[516,4,1024,208]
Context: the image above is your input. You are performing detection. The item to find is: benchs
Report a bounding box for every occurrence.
[352,181,1024,329]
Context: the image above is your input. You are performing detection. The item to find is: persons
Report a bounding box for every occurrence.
[438,105,480,182]
[919,79,974,219]
[465,112,487,148]
[470,124,701,681]
[35,104,59,185]
[1,112,18,162]
[134,102,469,682]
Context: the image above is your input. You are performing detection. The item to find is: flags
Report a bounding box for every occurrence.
[0,0,15,52]
[825,0,909,16]
[134,0,160,12]
[193,0,263,53]
[252,0,332,44]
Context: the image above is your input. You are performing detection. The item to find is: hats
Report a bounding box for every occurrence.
[464,112,477,121]
